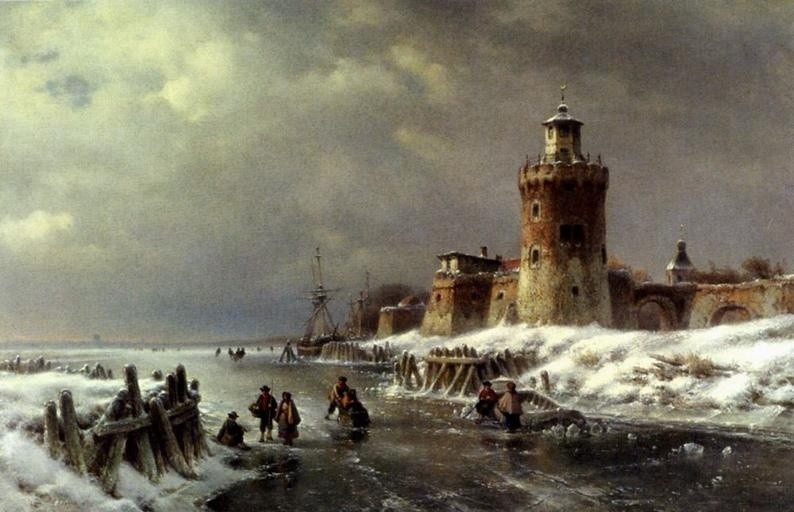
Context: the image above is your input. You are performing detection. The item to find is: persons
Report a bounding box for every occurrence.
[252,385,278,442]
[474,380,499,424]
[323,376,371,429]
[497,382,523,433]
[216,412,251,451]
[214,345,245,362]
[274,391,301,447]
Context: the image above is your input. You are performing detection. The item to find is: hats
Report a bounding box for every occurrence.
[259,384,271,393]
[482,380,493,387]
[338,376,347,382]
[227,410,239,418]
